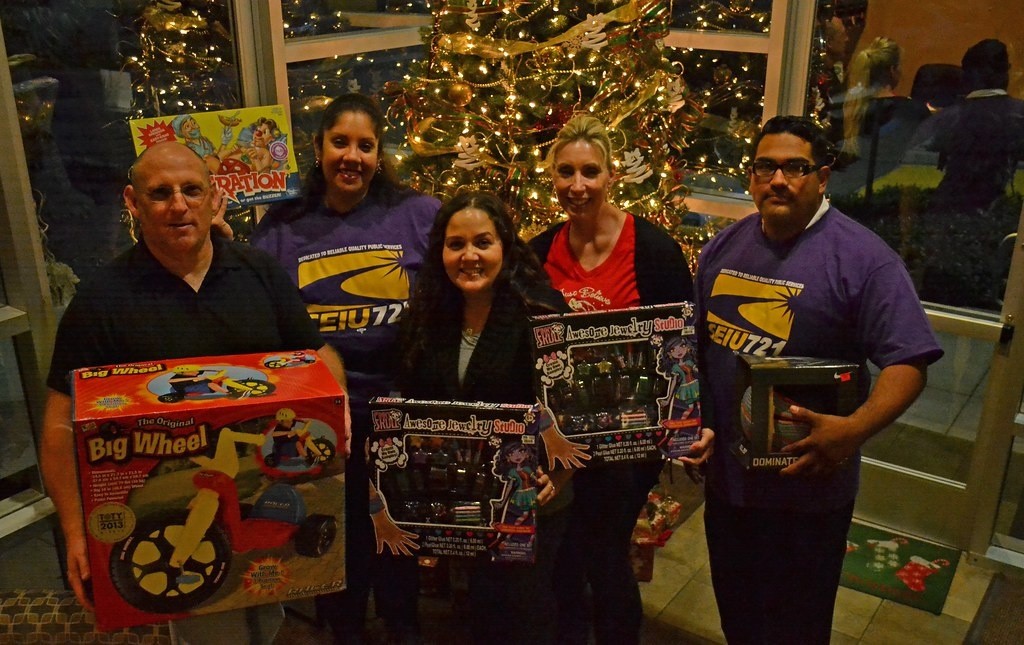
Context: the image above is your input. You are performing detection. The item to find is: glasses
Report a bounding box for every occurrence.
[753,158,818,178]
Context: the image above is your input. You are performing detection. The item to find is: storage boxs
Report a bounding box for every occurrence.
[368,395,542,568]
[71,348,348,633]
[524,301,702,471]
[731,352,867,473]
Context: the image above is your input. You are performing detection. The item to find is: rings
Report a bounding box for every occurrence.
[549,484,555,491]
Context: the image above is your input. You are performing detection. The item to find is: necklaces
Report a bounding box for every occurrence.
[322,182,369,210]
[463,320,481,336]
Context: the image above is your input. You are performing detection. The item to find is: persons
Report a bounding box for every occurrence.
[895,36,1023,314]
[242,92,442,645]
[701,113,945,645]
[37,139,354,645]
[827,33,933,170]
[381,193,578,645]
[520,111,716,645]
[814,14,849,148]
